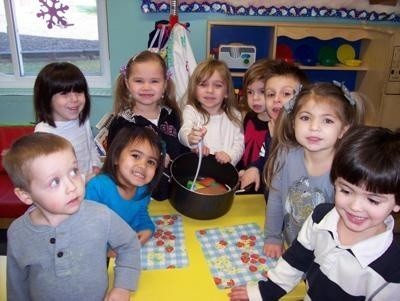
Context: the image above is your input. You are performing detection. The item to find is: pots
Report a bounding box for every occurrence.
[161,150,254,221]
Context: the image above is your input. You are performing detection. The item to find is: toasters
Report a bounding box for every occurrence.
[218,43,258,70]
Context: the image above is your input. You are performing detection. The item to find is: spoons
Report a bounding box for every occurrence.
[189,118,203,190]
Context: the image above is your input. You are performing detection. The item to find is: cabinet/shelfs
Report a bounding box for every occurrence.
[207,22,400,129]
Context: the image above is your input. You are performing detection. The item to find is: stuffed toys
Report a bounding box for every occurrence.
[209,46,218,63]
[233,88,248,111]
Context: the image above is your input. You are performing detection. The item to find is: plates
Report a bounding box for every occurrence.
[277,44,364,68]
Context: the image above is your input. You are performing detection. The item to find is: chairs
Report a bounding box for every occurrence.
[0,122,39,232]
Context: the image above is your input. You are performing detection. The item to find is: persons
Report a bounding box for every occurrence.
[239,63,308,194]
[178,61,245,169]
[107,51,210,202]
[85,125,162,257]
[237,58,286,173]
[33,62,105,186]
[228,126,400,301]
[2,131,141,300]
[262,83,376,259]
